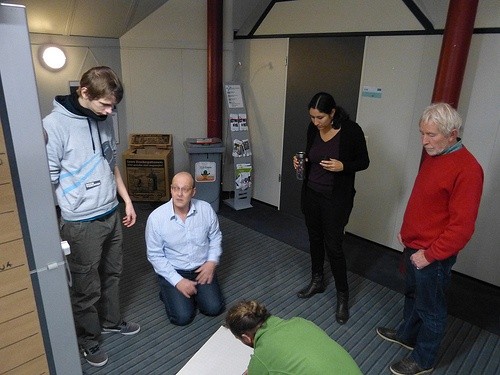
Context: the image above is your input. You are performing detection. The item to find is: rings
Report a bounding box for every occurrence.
[417,267,419,269]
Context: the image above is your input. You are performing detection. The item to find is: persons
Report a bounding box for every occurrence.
[43,66,140,366]
[225,298,362,375]
[293,93,369,324]
[376,102,484,375]
[145,172,225,326]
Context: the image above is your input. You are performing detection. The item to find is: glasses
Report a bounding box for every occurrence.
[171,185,193,193]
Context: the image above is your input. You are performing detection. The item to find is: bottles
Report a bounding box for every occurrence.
[295,151,307,180]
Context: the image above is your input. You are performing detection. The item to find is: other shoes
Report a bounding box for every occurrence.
[376,326,416,351]
[101,320,140,336]
[80,344,108,367]
[390,355,434,375]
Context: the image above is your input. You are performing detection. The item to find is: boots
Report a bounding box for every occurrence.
[297,273,326,298]
[335,290,349,325]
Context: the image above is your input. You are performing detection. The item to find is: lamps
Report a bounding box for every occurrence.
[38,43,67,72]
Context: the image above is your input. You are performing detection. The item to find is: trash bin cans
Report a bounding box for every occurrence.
[183,137,226,214]
